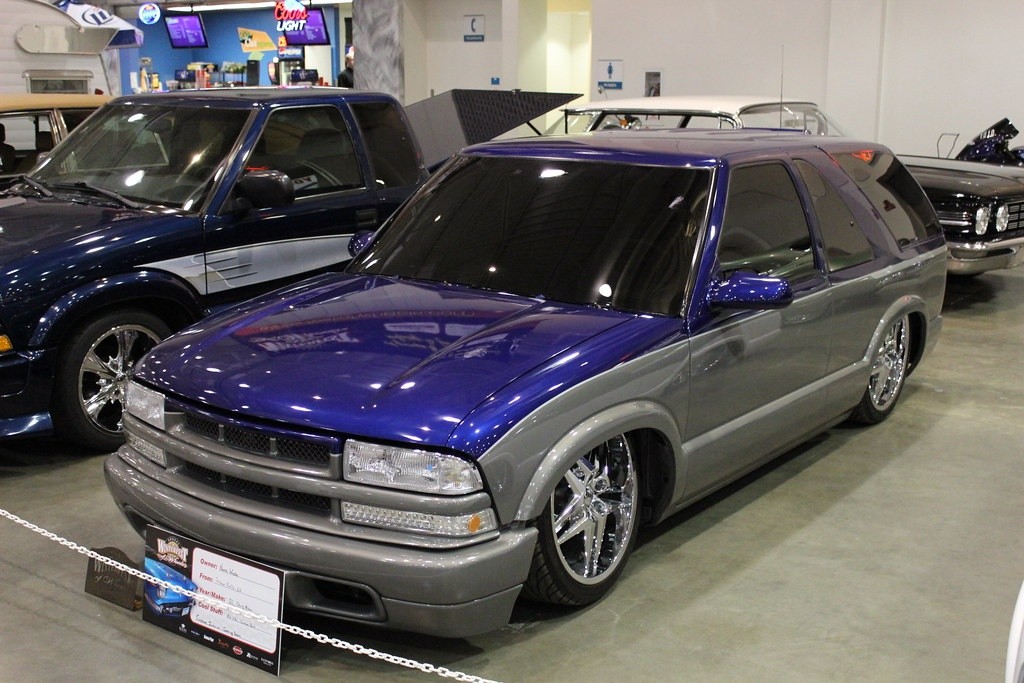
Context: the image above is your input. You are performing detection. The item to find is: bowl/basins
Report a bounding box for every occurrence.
[165,80,179,91]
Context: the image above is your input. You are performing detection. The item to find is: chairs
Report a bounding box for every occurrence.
[295,127,347,184]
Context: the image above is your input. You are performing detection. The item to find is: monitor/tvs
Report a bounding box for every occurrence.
[281,6,331,46]
[165,11,209,49]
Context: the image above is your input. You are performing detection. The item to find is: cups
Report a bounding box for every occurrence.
[315,77,328,86]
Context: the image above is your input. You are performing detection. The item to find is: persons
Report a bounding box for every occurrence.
[338,52,354,90]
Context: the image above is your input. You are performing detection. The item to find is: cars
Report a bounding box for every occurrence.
[499,94,1024,282]
[104,126,951,641]
[1,94,154,186]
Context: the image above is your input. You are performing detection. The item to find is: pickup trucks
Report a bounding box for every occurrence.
[1,84,445,456]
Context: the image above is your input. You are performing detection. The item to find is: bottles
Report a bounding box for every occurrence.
[140,66,159,92]
[197,69,210,88]
